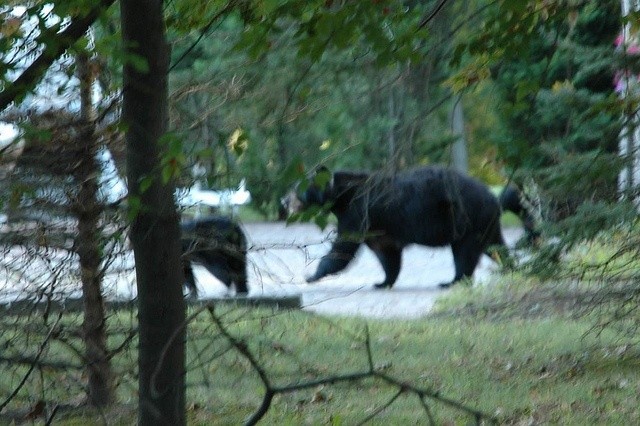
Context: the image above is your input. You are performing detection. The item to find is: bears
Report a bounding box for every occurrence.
[180,218,250,300]
[281,165,513,288]
